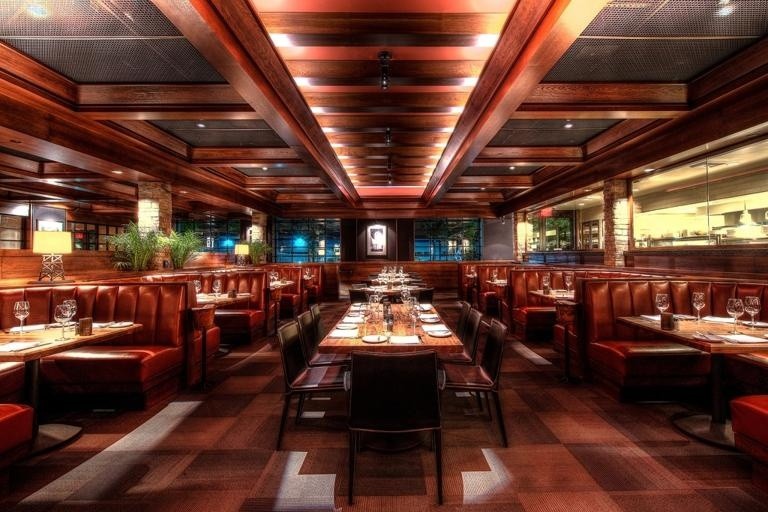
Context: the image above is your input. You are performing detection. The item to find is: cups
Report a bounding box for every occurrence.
[74,317,92,337]
[660,312,678,332]
[490,274,495,281]
[656,292,670,312]
[543,283,550,294]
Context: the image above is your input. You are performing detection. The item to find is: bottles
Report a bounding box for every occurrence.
[383,295,394,333]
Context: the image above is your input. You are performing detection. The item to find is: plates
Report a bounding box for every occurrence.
[347,302,362,318]
[416,303,440,324]
[109,322,134,328]
[362,335,388,343]
[428,330,452,338]
[692,332,723,344]
[336,323,357,330]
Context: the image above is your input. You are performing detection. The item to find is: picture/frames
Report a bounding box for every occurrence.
[363,221,390,259]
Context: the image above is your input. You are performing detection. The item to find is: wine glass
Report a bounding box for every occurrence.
[269,272,278,286]
[377,265,406,289]
[193,279,201,299]
[305,266,311,280]
[565,276,572,296]
[212,279,221,300]
[742,296,762,333]
[726,297,744,335]
[692,292,705,334]
[52,300,77,341]
[542,276,547,291]
[359,288,383,335]
[396,287,419,336]
[13,301,30,341]
